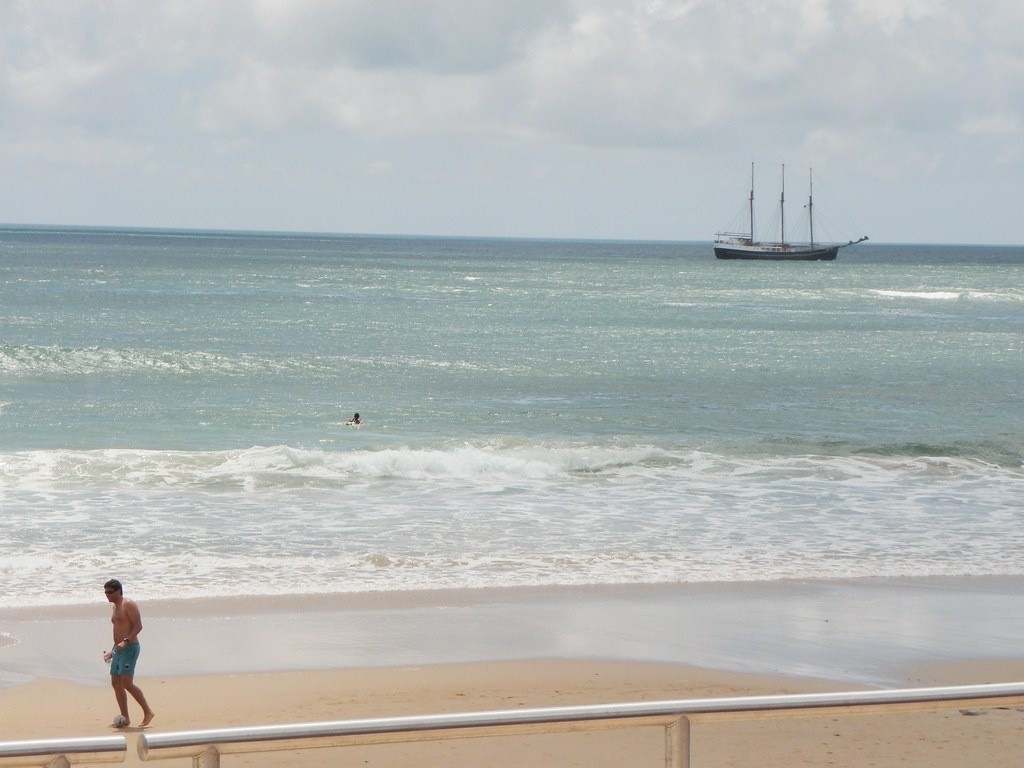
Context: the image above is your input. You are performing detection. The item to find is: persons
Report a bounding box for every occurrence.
[348,413,361,424]
[103,579,155,727]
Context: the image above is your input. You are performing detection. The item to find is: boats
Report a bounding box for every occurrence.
[713,162,869,262]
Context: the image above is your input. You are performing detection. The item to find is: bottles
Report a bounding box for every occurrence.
[104,651,111,665]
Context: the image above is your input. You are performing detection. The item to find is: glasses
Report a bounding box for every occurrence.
[105,589,119,594]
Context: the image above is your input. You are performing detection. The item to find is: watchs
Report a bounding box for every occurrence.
[124,638,130,645]
